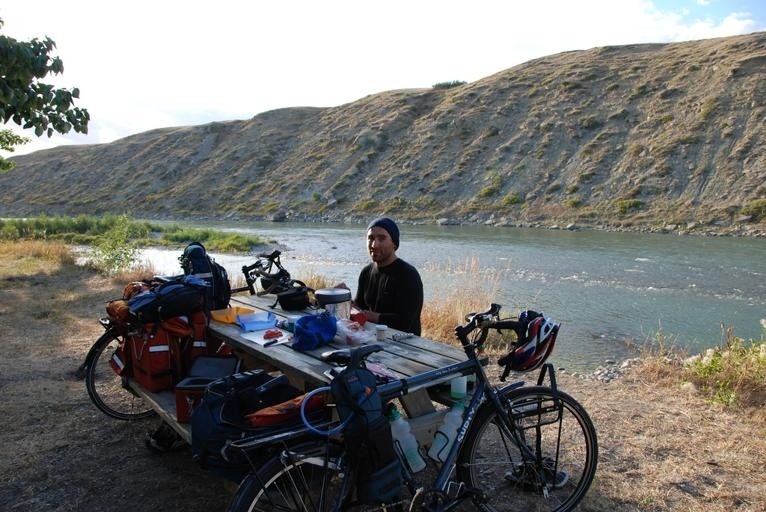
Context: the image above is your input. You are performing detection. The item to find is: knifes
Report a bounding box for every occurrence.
[262,333,289,348]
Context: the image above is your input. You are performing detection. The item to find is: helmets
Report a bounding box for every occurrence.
[498,310,561,372]
[260,269,290,294]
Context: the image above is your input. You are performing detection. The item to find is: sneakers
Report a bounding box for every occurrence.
[150,420,187,452]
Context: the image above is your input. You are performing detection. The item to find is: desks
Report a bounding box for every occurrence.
[184,294,489,471]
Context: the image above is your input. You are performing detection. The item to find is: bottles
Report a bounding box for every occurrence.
[288,315,301,332]
[376,325,388,342]
[451,375,467,400]
[429,404,466,462]
[387,409,427,473]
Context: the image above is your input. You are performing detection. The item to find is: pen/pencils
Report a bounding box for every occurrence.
[264,340,277,347]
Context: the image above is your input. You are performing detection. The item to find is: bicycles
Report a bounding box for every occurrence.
[76,251,315,420]
[221,302,599,510]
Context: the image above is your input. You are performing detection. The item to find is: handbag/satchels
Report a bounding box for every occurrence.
[190,369,290,481]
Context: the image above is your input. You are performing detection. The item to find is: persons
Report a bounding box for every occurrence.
[332,216,423,340]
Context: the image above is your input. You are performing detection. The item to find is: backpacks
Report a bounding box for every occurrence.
[127,241,231,323]
[277,279,315,310]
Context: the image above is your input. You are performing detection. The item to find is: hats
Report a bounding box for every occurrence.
[367,217,399,251]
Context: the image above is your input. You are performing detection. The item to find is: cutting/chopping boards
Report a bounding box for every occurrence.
[240,328,294,347]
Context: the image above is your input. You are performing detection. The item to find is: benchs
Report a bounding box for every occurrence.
[430,388,498,424]
[121,377,211,446]
